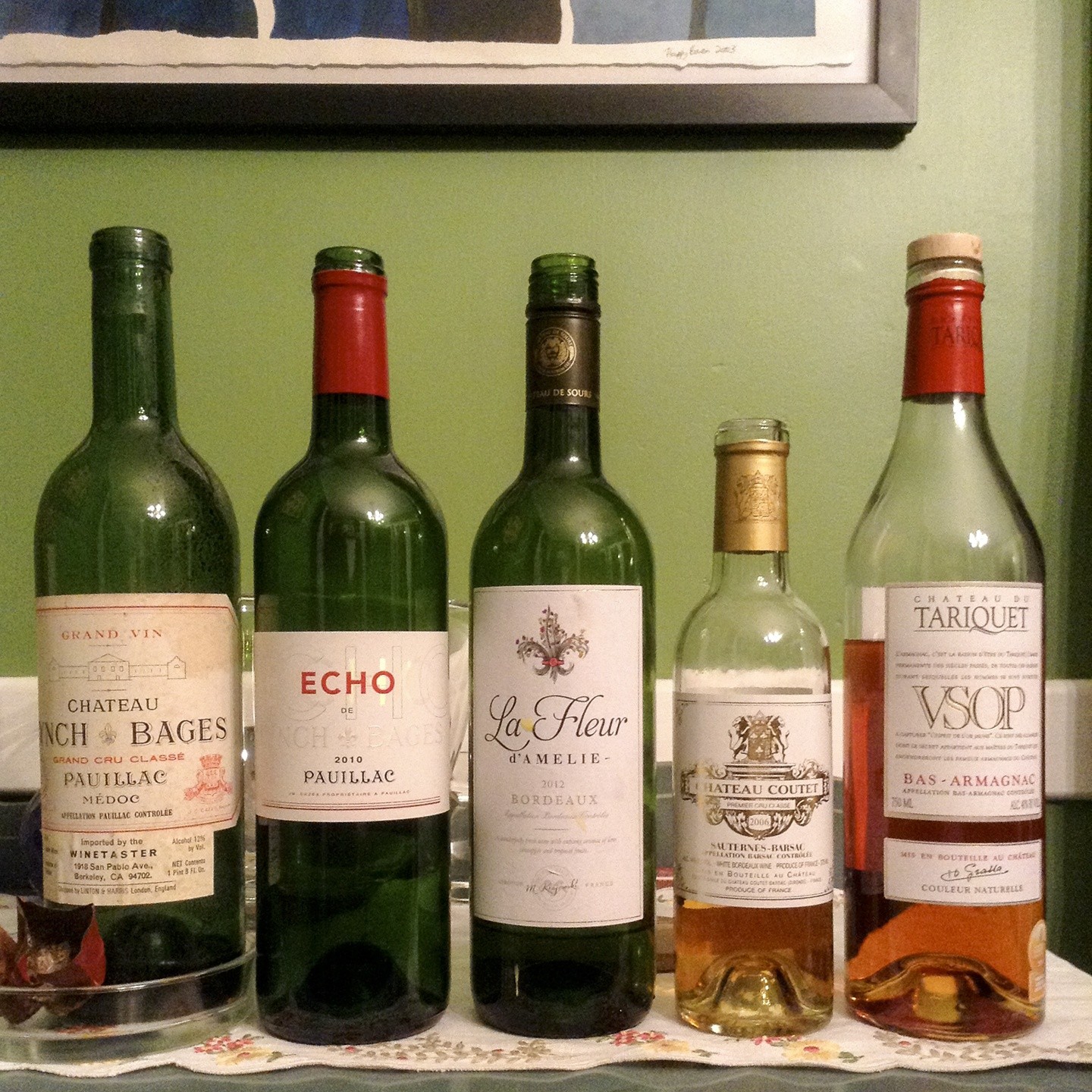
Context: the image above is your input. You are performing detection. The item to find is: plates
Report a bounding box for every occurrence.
[1,942,255,1023]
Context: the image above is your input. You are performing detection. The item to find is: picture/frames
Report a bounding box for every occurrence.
[0,0,929,140]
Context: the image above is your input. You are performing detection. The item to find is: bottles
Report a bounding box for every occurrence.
[32,224,245,1021]
[671,413,834,1035]
[254,244,451,1048]
[467,249,658,1038]
[844,231,1049,1041]
